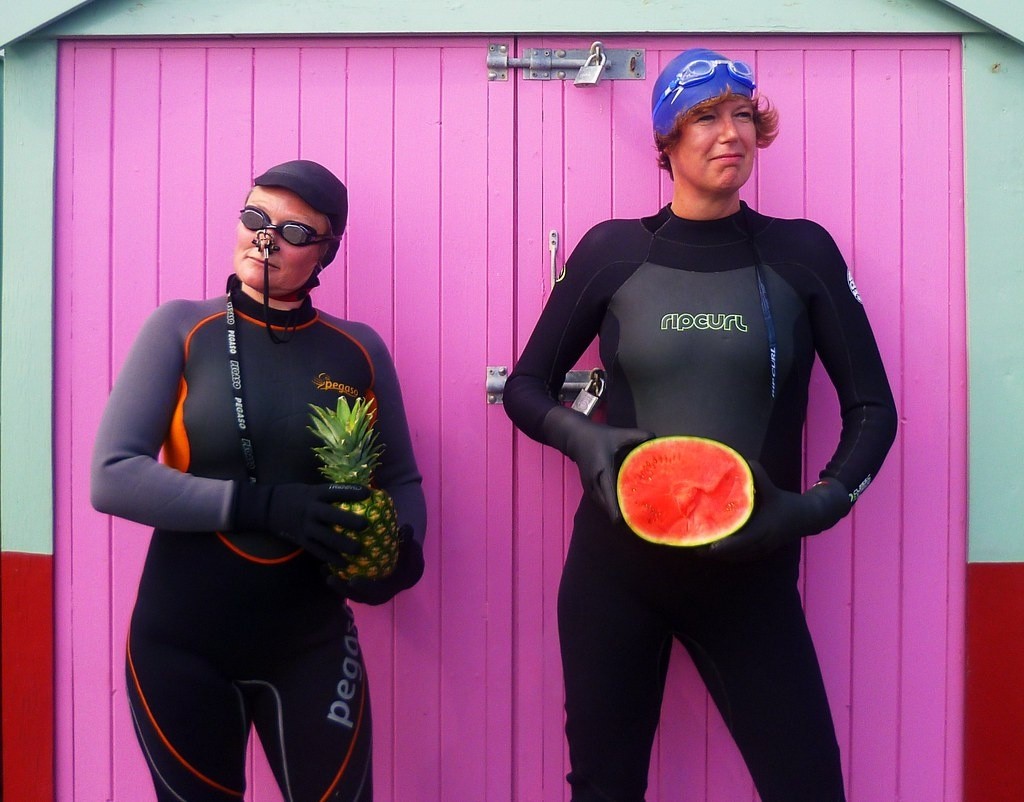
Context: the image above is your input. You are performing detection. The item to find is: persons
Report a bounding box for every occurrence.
[502,48,898,802]
[91,160,427,801]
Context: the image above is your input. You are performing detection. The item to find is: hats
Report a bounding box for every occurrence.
[244,160,348,283]
[652,48,751,135]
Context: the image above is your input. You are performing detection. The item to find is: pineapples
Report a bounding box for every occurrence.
[305,395,399,582]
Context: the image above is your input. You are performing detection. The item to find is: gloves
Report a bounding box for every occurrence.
[536,406,657,524]
[695,459,851,565]
[232,480,370,567]
[320,524,424,606]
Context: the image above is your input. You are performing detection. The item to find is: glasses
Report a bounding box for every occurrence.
[676,60,756,89]
[239,206,318,246]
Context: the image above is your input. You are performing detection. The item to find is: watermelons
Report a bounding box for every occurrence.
[616,435,755,546]
